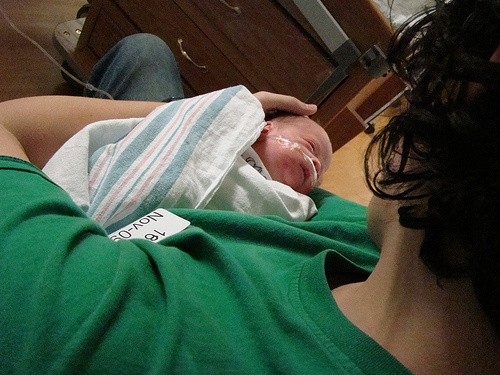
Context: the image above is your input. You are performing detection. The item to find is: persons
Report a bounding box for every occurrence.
[1,0,500,374]
[241,108,334,196]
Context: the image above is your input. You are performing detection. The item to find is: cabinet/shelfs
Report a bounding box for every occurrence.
[53,0,439,159]
